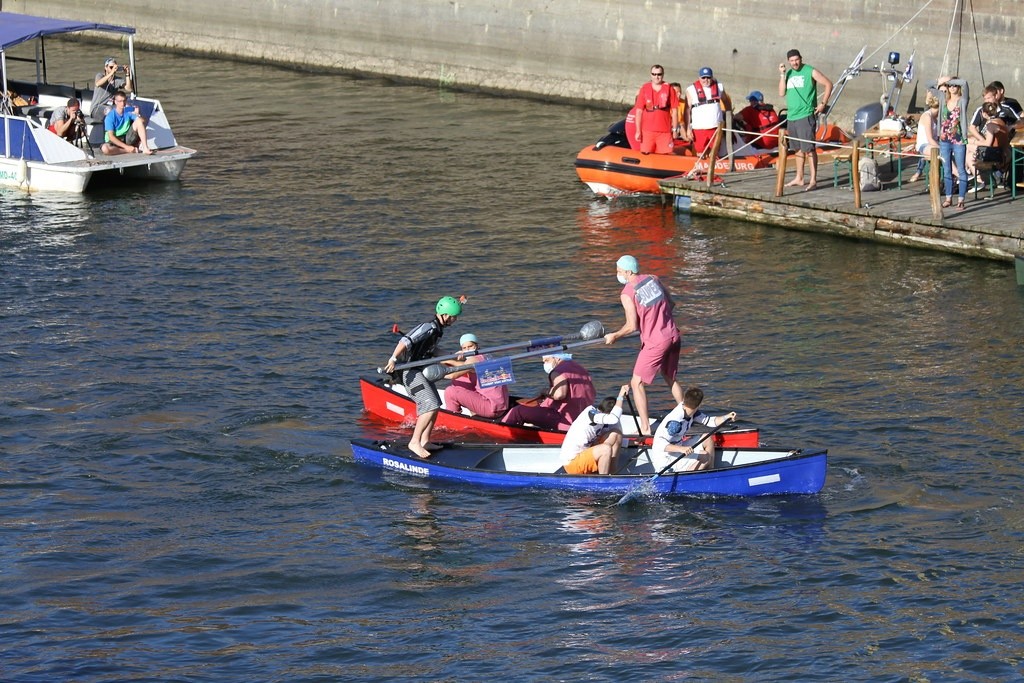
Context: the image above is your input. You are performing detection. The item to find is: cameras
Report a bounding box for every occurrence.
[75,110,84,119]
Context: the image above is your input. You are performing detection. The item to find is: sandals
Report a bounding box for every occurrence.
[958,202,964,208]
[941,201,952,208]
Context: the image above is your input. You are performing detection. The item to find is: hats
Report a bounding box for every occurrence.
[699,67,713,78]
[68,98,79,107]
[105,58,117,65]
[787,50,802,58]
[746,91,763,101]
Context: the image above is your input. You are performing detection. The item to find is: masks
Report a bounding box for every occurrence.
[617,271,629,284]
[464,347,478,360]
[543,359,554,374]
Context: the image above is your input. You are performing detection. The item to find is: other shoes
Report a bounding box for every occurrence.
[910,175,919,183]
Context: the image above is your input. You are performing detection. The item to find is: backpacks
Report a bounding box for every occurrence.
[858,157,883,192]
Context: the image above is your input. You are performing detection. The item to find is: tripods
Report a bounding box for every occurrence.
[76,121,94,157]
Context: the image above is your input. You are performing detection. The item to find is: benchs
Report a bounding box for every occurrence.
[471,396,522,422]
[918,156,1007,200]
[8,104,105,144]
[656,465,673,474]
[553,465,568,474]
[831,127,874,190]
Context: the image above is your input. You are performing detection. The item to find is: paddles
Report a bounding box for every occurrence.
[516,392,545,407]
[624,392,642,438]
[392,323,405,336]
[617,418,732,505]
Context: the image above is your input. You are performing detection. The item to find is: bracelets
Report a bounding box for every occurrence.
[973,140,979,145]
[391,356,398,362]
[125,73,130,76]
[671,127,679,132]
[136,114,142,119]
[821,102,827,107]
[780,74,784,77]
[723,414,729,424]
[616,396,624,400]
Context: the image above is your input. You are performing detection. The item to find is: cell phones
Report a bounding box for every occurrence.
[117,67,124,71]
[124,106,135,112]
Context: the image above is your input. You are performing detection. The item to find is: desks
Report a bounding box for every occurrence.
[861,113,922,191]
[1009,111,1024,199]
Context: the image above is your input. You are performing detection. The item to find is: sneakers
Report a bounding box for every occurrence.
[996,178,1007,188]
[968,183,986,193]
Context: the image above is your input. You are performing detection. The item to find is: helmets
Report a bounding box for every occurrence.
[436,297,463,316]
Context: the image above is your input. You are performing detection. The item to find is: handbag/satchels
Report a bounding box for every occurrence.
[976,147,1003,162]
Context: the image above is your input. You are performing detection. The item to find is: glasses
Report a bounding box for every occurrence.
[109,66,113,70]
[940,90,947,93]
[947,85,957,87]
[651,73,662,76]
[701,77,711,79]
[116,99,127,103]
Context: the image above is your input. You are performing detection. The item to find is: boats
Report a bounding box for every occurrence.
[0,11,198,193]
[349,435,829,497]
[359,375,760,447]
[574,102,918,197]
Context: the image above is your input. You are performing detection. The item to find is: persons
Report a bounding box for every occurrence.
[559,384,630,474]
[634,64,678,155]
[625,82,689,152]
[651,387,737,472]
[778,50,833,192]
[906,76,1024,208]
[382,294,463,459]
[89,57,147,125]
[438,332,509,418]
[100,91,153,155]
[501,352,596,433]
[683,67,732,159]
[733,90,780,149]
[49,98,87,146]
[604,254,684,435]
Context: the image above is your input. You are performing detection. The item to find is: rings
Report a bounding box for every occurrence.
[391,369,394,372]
[610,342,613,345]
